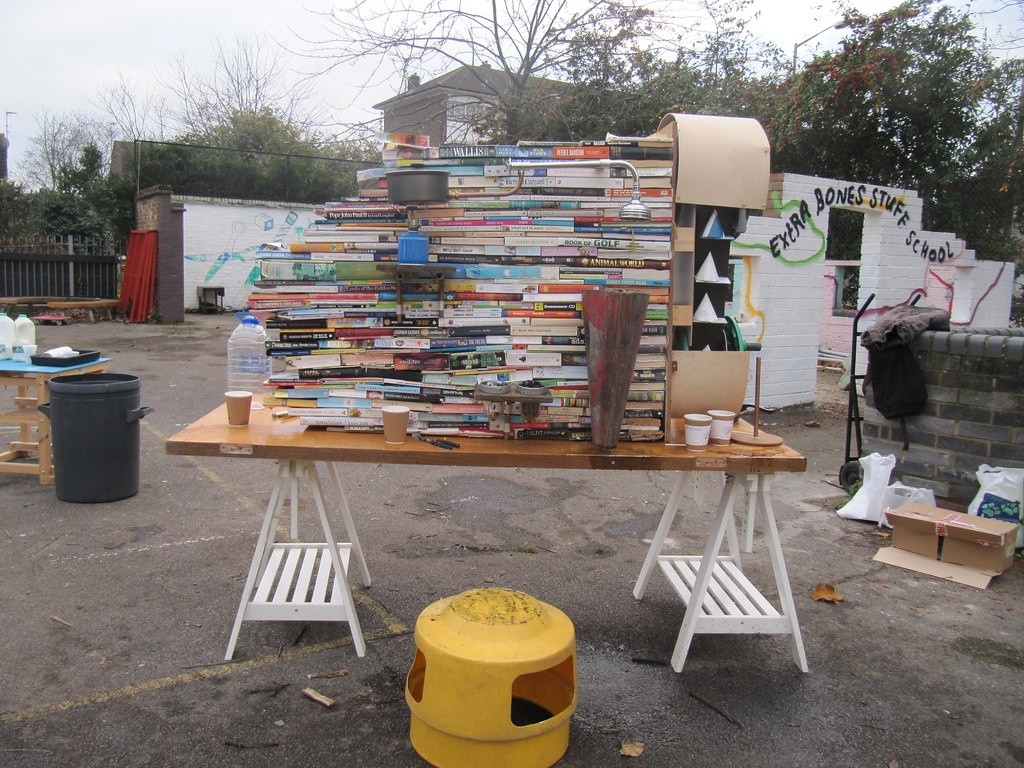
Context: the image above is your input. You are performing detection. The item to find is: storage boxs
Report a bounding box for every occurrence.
[872,502,1019,591]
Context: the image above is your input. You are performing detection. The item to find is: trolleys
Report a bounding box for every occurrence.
[839,292,923,495]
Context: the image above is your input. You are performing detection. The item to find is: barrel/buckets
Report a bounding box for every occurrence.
[38,373,151,504]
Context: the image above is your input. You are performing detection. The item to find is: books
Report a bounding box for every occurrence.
[246,133,673,443]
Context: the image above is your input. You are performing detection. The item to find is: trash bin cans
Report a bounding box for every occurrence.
[37,372,155,504]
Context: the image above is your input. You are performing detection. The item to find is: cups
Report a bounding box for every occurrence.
[382,405,410,444]
[23,345,37,364]
[708,409,738,444]
[225,391,253,427]
[683,413,712,452]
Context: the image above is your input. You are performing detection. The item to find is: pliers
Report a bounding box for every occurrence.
[419,436,460,450]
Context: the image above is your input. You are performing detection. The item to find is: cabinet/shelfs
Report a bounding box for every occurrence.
[644,121,750,447]
[0,355,112,484]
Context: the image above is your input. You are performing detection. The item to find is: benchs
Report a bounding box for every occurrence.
[0,297,121,322]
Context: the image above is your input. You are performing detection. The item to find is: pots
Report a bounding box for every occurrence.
[385,163,450,202]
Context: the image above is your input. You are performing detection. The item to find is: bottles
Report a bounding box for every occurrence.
[396,219,429,265]
[0,313,14,360]
[227,316,272,410]
[14,314,37,361]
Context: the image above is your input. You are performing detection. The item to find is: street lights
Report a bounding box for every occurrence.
[4,112,16,138]
[792,18,850,77]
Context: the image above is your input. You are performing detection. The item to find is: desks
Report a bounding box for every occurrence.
[197,285,224,314]
[166,391,810,673]
